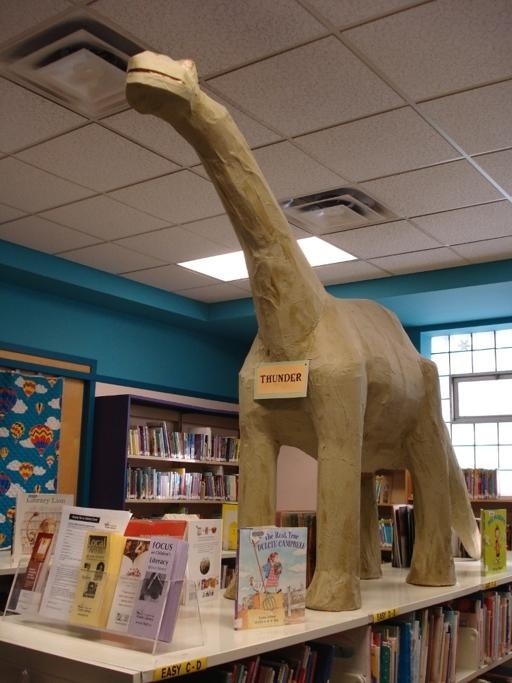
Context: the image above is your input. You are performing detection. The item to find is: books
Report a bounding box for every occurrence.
[15,492,316,640]
[376,469,507,576]
[371,584,512,683]
[225,640,338,682]
[129,420,243,464]
